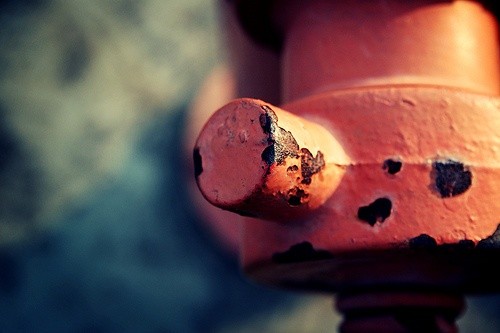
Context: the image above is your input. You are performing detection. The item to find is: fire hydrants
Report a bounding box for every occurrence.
[193,0,500,333]
